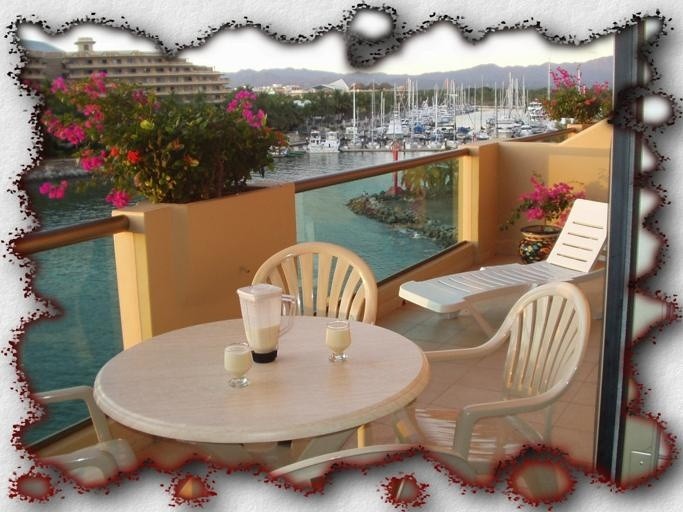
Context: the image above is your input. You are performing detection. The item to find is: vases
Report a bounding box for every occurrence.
[518,225,562,264]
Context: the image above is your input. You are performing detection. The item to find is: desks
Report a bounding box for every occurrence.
[89,311,433,465]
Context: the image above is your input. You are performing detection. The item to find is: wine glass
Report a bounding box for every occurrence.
[326,321,352,360]
[224,342,251,386]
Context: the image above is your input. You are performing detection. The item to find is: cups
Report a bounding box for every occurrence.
[238,284,297,362]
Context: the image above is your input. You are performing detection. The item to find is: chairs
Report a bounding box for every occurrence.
[19,387,139,491]
[403,281,590,481]
[399,199,608,337]
[256,441,475,508]
[246,243,378,327]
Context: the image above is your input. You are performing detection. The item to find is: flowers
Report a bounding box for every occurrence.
[535,63,613,126]
[496,170,587,231]
[29,68,294,212]
